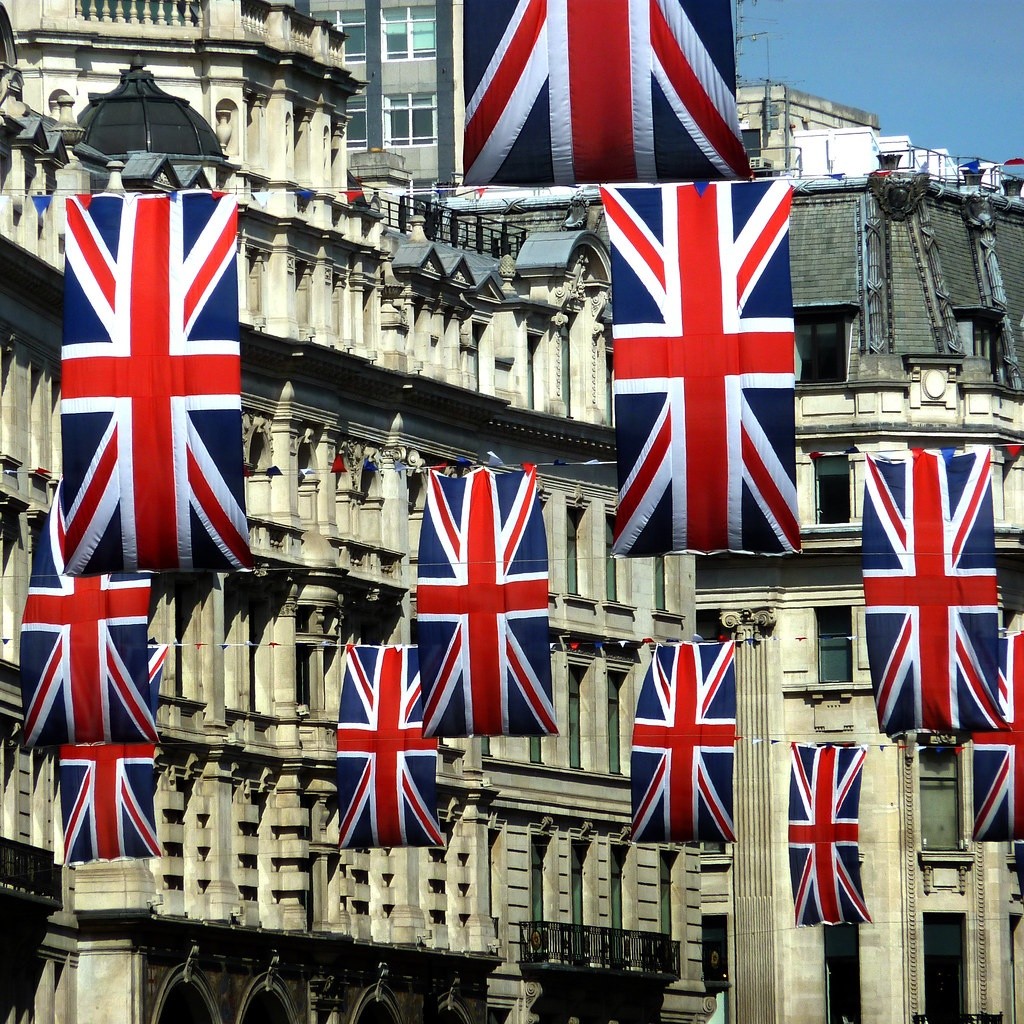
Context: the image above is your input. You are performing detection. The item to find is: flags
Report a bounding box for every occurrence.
[600,182,803,558]
[463,0,753,185]
[61,195,254,577]
[973,630,1024,842]
[416,467,558,737]
[18,485,159,748]
[337,647,444,850]
[788,745,873,928]
[56,645,169,861]
[862,448,1011,735]
[630,641,737,843]
